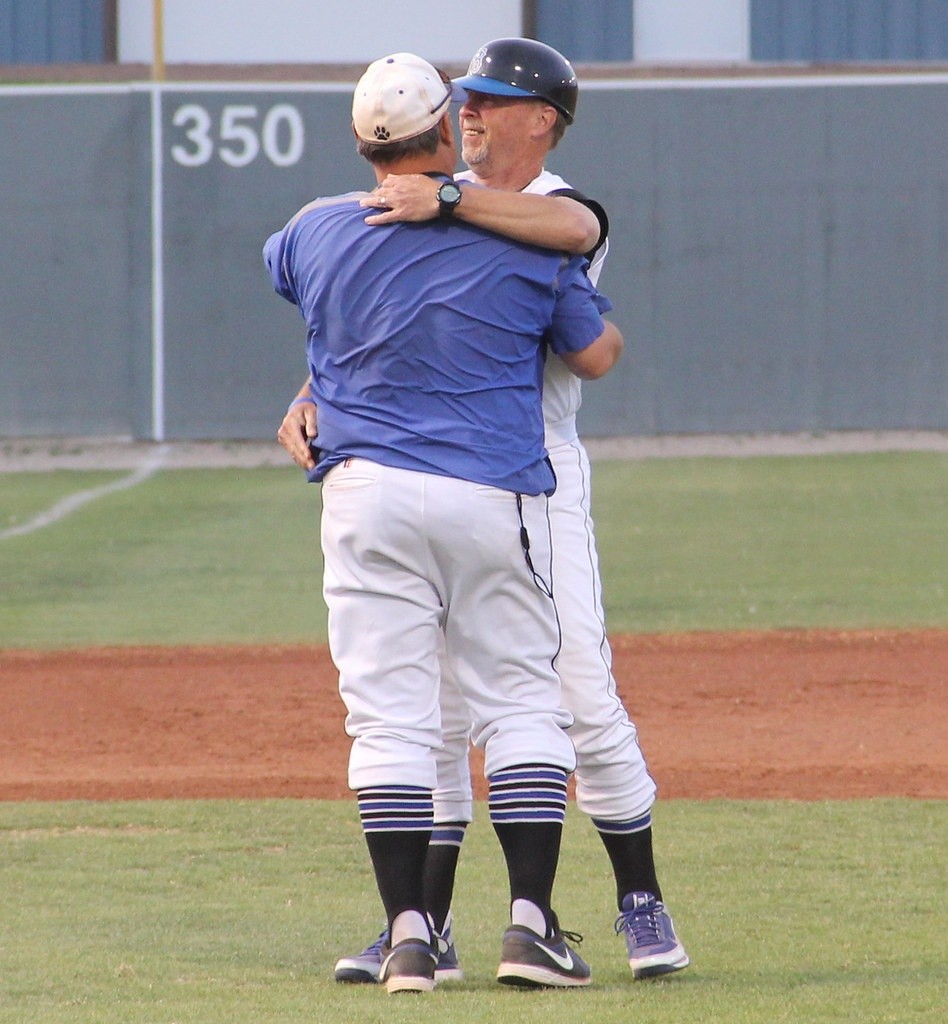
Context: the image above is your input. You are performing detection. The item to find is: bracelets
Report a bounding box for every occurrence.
[288,397,315,408]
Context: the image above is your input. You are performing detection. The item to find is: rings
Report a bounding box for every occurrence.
[381,198,386,207]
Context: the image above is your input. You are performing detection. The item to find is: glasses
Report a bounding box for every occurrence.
[429,65,454,114]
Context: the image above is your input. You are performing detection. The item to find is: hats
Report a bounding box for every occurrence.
[351,52,469,144]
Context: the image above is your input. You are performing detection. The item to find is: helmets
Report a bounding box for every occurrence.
[450,38,577,122]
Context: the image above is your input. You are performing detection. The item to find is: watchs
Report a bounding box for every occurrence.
[437,181,462,219]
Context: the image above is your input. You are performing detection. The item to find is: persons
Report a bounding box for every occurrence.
[279,37,688,978]
[263,52,626,992]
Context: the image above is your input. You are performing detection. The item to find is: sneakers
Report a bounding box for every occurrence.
[379,938,436,994]
[334,918,462,982]
[496,910,592,986]
[615,891,689,979]
